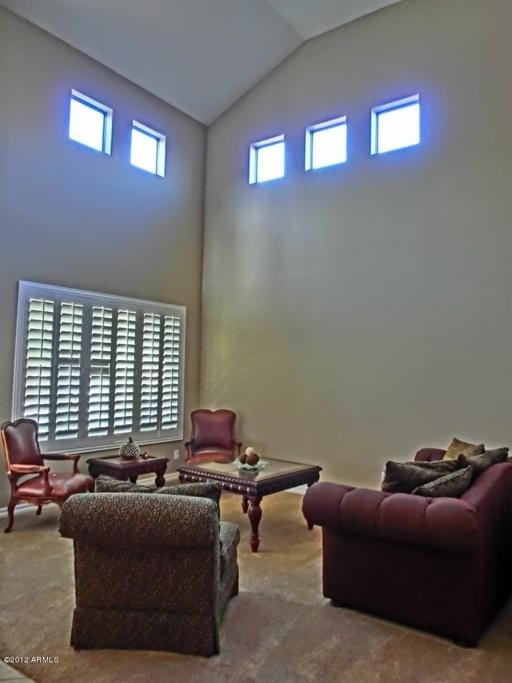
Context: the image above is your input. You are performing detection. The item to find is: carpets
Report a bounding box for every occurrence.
[1,490,512,682]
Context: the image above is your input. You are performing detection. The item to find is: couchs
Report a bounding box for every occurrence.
[302,448,512,648]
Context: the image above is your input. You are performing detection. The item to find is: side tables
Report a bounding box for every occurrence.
[86,453,171,488]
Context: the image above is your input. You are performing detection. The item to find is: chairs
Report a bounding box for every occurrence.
[57,474,240,657]
[0,416,93,535]
[182,406,243,463]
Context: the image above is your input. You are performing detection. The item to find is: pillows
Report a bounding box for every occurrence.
[377,436,510,500]
